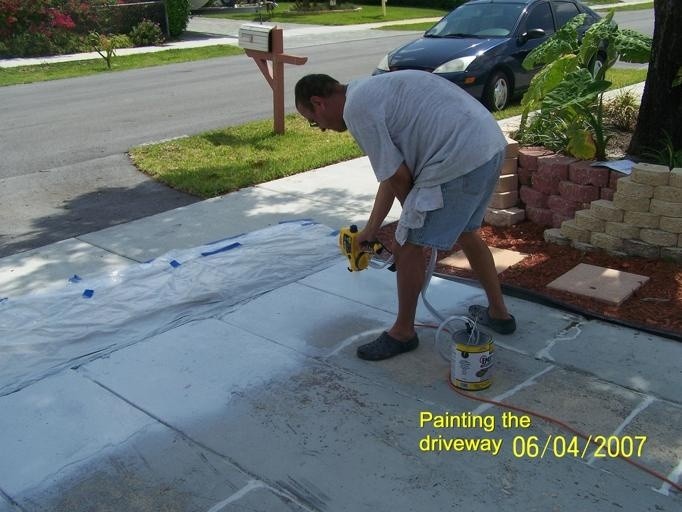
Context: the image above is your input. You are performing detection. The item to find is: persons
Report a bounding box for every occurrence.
[291,69,519,363]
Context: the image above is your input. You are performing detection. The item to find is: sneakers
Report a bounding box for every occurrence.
[468,304,516,335]
[356,329,420,362]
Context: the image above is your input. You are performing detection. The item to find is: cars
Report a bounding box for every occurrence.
[365,1,620,115]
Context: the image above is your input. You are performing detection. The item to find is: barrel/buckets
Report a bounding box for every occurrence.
[449,329,494,391]
